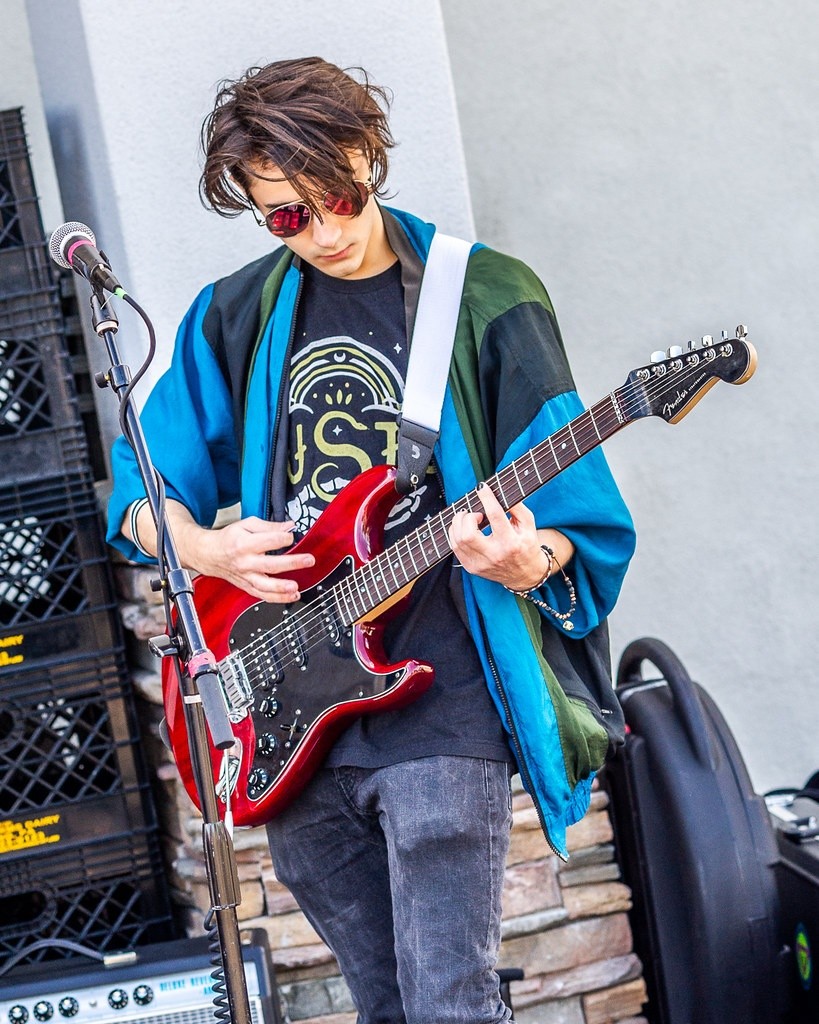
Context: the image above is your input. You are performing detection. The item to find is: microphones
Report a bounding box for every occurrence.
[50,222,123,295]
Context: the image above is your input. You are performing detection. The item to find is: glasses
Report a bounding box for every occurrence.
[248,167,373,238]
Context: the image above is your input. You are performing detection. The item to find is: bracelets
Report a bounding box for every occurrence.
[129,496,156,561]
[502,547,552,596]
[505,544,577,631]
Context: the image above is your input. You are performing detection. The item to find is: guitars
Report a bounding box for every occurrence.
[159,324,759,829]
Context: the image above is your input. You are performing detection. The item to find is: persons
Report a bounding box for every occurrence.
[100,52,637,1024]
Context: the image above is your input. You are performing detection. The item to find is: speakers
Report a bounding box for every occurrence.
[0,928,283,1024]
